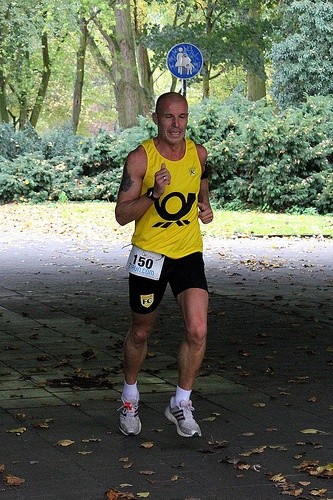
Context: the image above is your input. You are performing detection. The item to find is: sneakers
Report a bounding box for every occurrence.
[165,397,201,438]
[117,395,141,435]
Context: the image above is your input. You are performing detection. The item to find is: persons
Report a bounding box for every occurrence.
[116,91,214,440]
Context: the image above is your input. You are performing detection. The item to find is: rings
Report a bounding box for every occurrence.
[163,176,166,181]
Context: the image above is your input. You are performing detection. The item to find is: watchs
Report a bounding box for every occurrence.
[147,187,160,200]
